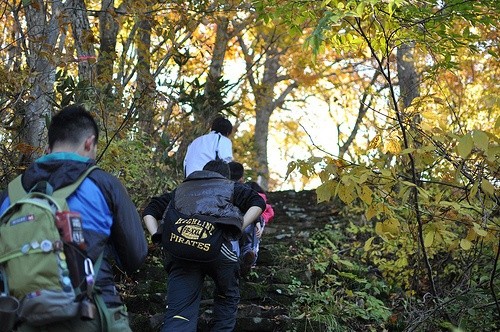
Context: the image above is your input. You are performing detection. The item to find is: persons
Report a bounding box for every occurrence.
[227,161,275,280]
[142,157,266,332]
[0,102,148,332]
[183,116,233,180]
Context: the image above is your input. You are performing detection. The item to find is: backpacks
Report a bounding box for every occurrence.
[0,166,104,325]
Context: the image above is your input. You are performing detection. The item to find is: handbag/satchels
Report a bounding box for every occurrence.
[162,203,224,261]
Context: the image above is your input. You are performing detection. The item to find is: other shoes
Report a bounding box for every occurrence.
[240,251,255,277]
